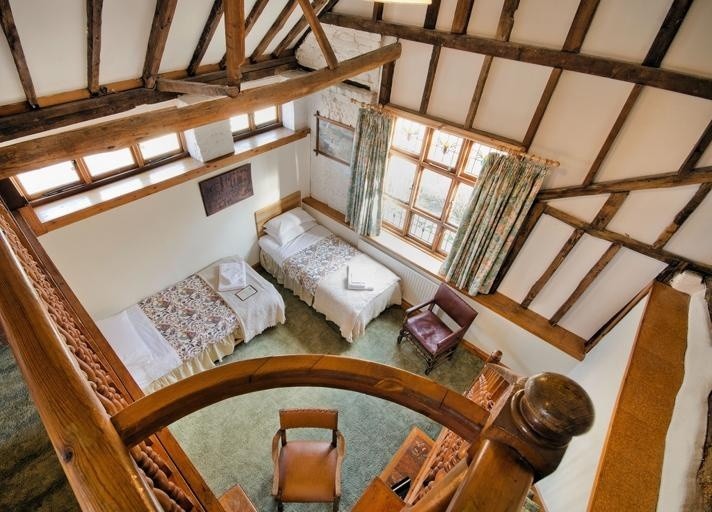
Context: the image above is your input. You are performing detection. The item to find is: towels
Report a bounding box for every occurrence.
[218,260,247,291]
[347,264,376,291]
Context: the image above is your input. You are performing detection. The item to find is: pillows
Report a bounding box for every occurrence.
[96,310,153,371]
[262,206,318,247]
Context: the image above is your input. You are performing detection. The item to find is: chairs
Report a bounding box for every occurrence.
[271,407,347,512]
[397,283,479,375]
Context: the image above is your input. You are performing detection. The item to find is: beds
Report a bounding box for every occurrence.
[96,254,287,396]
[254,190,402,343]
